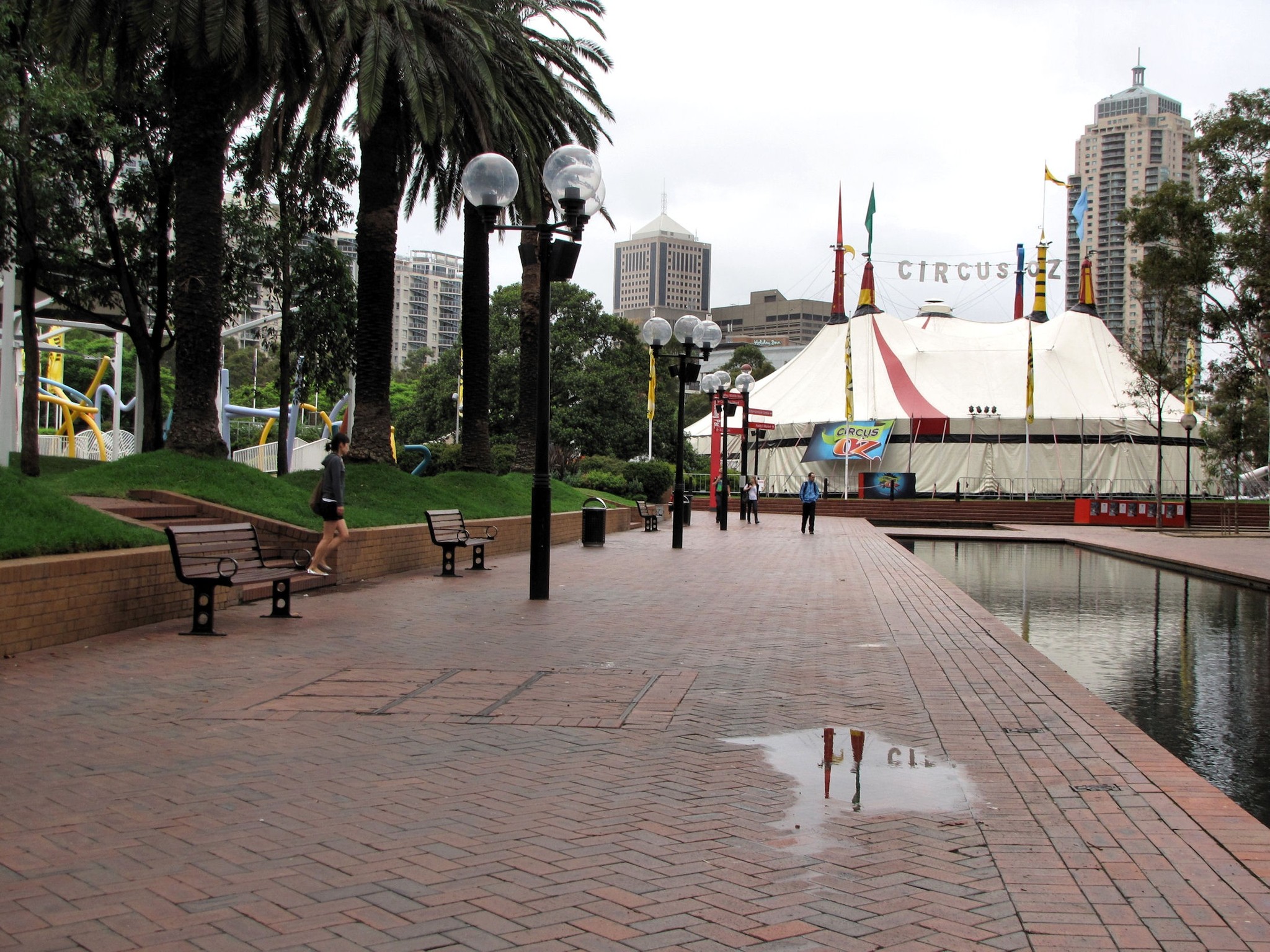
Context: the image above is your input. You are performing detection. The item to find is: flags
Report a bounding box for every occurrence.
[1046,166,1075,189]
[1071,186,1087,242]
[863,183,875,255]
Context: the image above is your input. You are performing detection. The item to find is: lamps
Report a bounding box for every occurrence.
[992,407,997,413]
[969,406,973,413]
[976,406,981,413]
[984,406,989,413]
[1088,249,1097,256]
[862,252,869,256]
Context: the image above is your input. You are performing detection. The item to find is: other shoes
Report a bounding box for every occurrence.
[307,568,329,576]
[756,522,759,524]
[716,519,719,523]
[311,557,332,570]
[748,521,751,523]
[802,527,805,533]
[810,530,813,534]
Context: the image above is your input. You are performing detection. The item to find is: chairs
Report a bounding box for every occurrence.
[636,501,662,532]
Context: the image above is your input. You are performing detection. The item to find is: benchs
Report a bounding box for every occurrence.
[164,521,313,637]
[424,508,497,577]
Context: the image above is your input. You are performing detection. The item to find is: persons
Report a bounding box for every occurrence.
[743,475,760,524]
[711,468,731,524]
[799,472,819,535]
[306,432,349,575]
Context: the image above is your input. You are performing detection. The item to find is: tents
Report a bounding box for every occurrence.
[683,315,1226,499]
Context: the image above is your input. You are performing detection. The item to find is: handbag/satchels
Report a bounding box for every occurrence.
[309,477,322,516]
[742,483,750,502]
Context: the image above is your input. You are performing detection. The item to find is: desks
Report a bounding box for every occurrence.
[858,473,916,499]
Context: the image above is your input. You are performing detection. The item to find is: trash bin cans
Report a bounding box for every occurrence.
[685,495,693,525]
[682,495,690,526]
[581,497,607,547]
